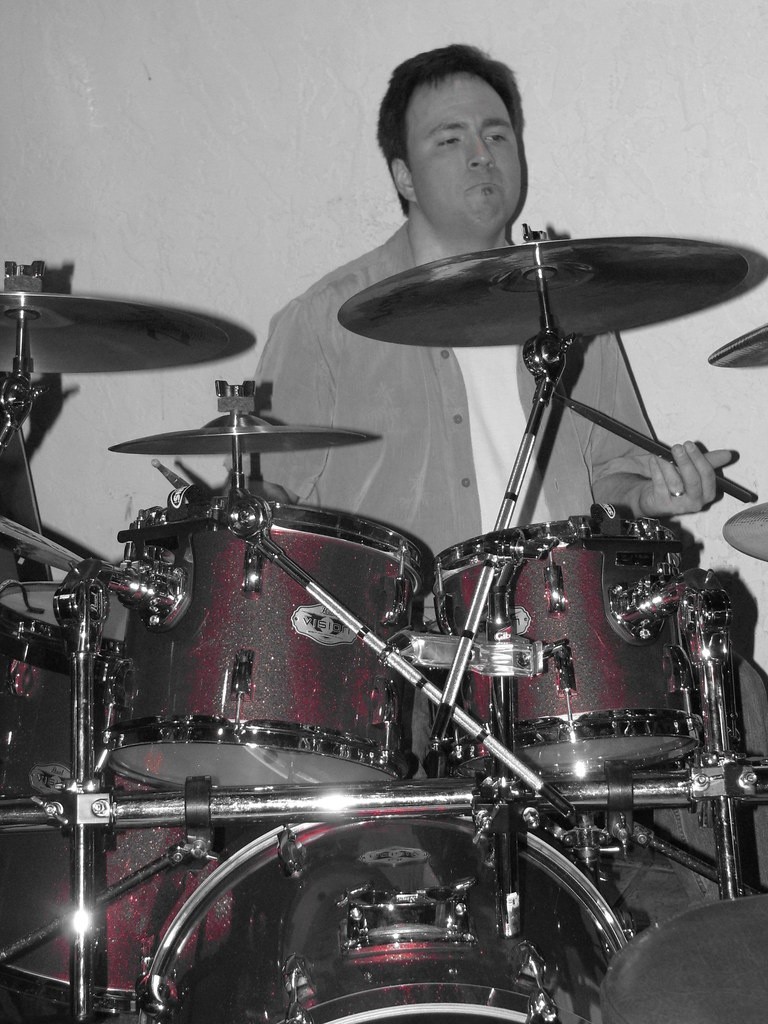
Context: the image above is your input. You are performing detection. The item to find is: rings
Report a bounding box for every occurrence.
[669,490,686,498]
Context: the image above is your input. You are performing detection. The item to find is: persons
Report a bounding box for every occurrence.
[197,45,738,784]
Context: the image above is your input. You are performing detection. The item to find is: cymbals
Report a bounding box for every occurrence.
[106,417,381,457]
[709,321,766,370]
[722,503,768,563]
[1,291,255,374]
[0,514,92,576]
[338,234,768,348]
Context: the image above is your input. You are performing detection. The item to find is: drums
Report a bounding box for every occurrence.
[140,807,631,1024]
[108,504,433,793]
[0,577,241,1007]
[434,512,706,783]
